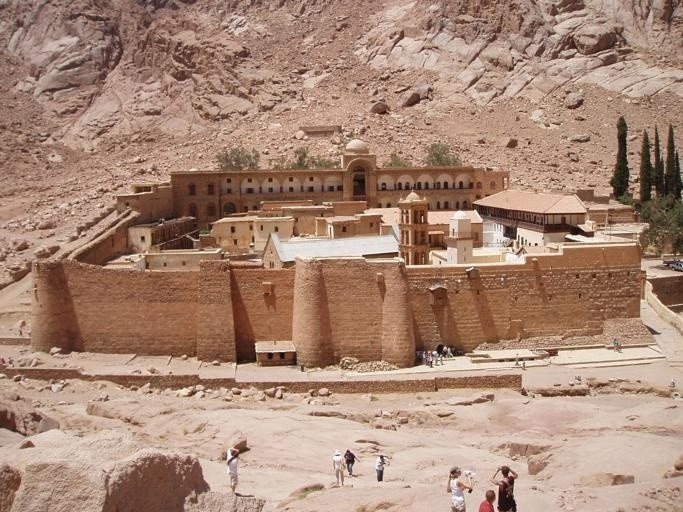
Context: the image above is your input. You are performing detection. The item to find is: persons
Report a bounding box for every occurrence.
[446,465,472,511]
[332,449,345,485]
[490,465,517,512]
[613,338,622,353]
[375,454,389,481]
[343,448,360,475]
[416,344,455,368]
[479,489,496,512]
[514,353,521,365]
[226,447,239,492]
